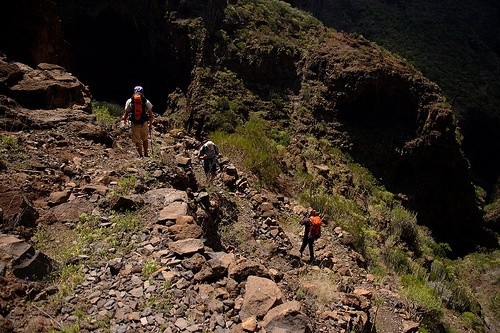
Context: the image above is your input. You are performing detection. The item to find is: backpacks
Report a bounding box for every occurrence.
[132,93,147,125]
[307,216,321,238]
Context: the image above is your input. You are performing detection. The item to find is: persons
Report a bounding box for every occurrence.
[196,140,219,188]
[297,209,323,265]
[123,85,155,158]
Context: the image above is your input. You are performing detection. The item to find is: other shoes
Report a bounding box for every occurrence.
[307,259,313,265]
[139,153,147,158]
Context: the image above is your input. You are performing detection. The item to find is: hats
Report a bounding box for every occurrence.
[133,86,144,93]
[309,209,315,216]
